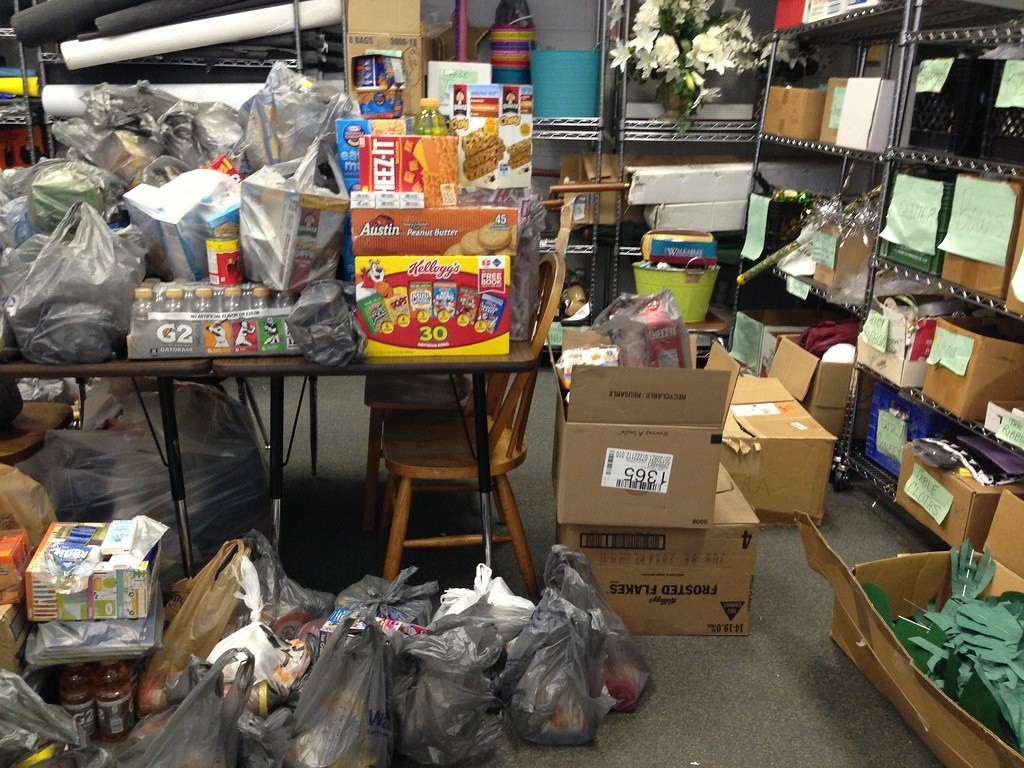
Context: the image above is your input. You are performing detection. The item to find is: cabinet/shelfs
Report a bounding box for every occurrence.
[834,0,1024,552]
[613,0,864,300]
[729,0,915,480]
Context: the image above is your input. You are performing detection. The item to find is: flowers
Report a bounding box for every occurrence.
[608,0,808,133]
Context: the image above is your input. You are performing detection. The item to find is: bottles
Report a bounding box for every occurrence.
[411,98,450,136]
[53,660,136,743]
[129,275,296,333]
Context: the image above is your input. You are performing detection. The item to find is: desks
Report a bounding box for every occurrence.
[0,332,537,578]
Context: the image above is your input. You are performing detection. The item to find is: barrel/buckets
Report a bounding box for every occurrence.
[528,38,603,116]
[490,25,533,84]
[631,259,719,324]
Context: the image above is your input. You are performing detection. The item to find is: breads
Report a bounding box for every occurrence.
[461,125,532,181]
[443,223,518,259]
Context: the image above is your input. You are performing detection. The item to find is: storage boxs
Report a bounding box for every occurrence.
[551,150,1024,636]
[908,56,991,157]
[0,528,32,604]
[774,0,883,30]
[342,0,608,357]
[25,521,166,622]
[975,57,1024,164]
[318,609,428,658]
[124,59,535,356]
[0,603,32,673]
[834,77,916,153]
[626,101,753,120]
[763,85,826,140]
[791,489,1024,768]
[818,76,848,143]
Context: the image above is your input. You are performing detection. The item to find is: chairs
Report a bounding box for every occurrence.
[0,402,74,466]
[380,251,566,597]
[360,366,506,532]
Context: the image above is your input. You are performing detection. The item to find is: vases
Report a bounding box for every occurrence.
[658,75,680,122]
